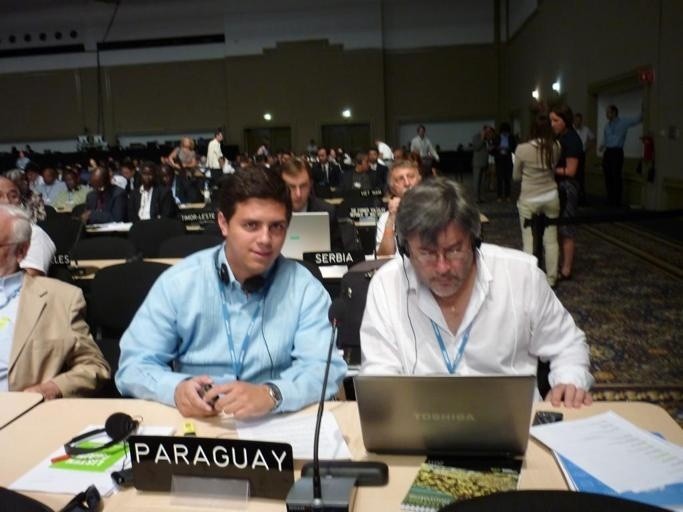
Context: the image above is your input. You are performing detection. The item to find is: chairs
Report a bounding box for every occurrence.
[337,272,369,362]
[52,267,119,367]
[337,218,355,250]
[124,217,192,259]
[57,217,86,253]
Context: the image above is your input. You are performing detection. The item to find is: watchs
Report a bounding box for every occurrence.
[268,383,283,407]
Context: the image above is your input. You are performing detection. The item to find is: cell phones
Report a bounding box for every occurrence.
[197,383,220,407]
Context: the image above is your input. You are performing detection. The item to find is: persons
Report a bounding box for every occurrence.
[358,174,597,407]
[599,103,643,211]
[1,204,114,401]
[572,111,595,155]
[115,163,349,420]
[411,122,439,174]
[470,103,584,287]
[1,103,405,223]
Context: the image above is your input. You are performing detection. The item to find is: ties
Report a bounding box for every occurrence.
[323,165,327,183]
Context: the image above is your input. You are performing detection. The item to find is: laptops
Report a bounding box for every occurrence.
[352,374,533,456]
[280,212,331,261]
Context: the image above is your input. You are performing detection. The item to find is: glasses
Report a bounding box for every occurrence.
[406,240,471,264]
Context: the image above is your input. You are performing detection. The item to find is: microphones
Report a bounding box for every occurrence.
[285,300,356,510]
[369,198,385,260]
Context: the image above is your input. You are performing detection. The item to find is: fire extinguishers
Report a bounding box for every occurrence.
[639,136,651,160]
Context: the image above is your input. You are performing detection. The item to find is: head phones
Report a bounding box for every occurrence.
[65,413,137,457]
[212,244,282,295]
[392,207,484,258]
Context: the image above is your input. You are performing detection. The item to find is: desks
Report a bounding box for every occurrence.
[141,254,401,279]
[176,197,344,209]
[0,390,47,430]
[59,257,132,281]
[88,210,489,236]
[0,392,681,512]
